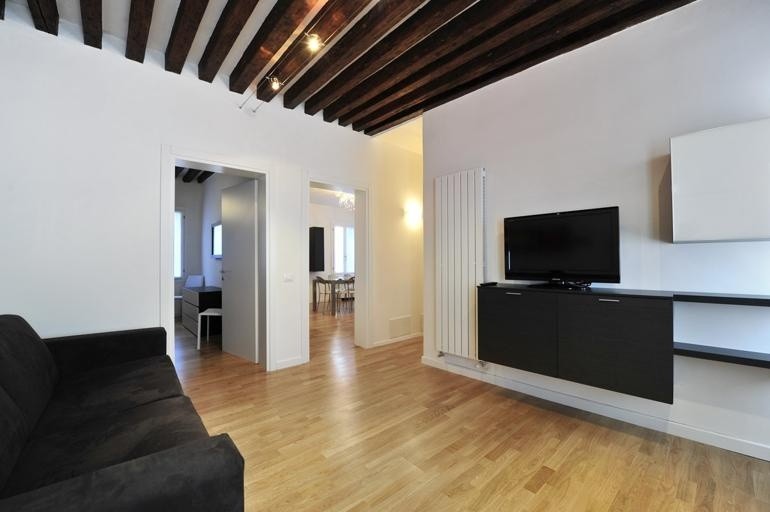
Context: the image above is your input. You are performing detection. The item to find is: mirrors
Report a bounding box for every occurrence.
[209,220,222,261]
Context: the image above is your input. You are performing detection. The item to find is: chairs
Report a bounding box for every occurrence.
[311,274,355,318]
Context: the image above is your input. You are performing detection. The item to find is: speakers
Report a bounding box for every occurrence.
[309,226,325,273]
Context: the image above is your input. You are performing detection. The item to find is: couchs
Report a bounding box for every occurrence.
[0,314,247,510]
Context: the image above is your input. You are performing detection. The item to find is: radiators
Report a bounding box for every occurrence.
[430,164,488,365]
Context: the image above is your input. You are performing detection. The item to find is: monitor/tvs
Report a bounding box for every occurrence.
[501,205,622,289]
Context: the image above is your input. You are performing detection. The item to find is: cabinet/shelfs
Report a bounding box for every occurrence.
[477,281,769,408]
[180,285,222,338]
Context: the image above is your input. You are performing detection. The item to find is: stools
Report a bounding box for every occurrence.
[196,307,222,352]
